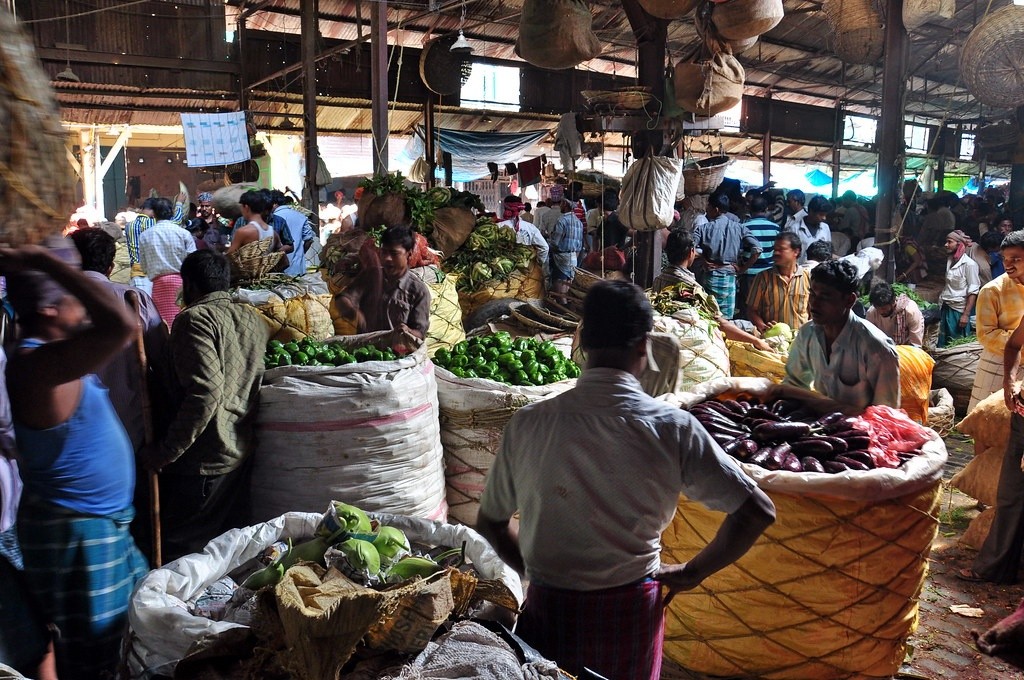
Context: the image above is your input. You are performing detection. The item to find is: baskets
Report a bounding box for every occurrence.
[680,130,732,195]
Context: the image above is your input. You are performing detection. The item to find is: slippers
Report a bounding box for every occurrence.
[957,567,986,582]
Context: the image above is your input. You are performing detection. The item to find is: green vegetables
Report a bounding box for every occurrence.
[647,283,721,338]
[859,281,926,309]
[245,276,302,290]
[357,169,537,290]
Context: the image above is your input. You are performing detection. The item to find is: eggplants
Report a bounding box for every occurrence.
[688,395,920,472]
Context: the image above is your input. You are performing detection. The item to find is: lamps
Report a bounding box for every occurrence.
[866,62,881,109]
[57,0,79,82]
[447,0,476,53]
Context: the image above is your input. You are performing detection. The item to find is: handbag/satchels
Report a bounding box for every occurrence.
[619,147,683,229]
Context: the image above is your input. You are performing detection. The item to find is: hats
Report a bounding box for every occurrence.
[197,192,214,203]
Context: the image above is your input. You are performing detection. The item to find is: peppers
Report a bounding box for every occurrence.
[939,334,978,348]
[262,334,409,368]
[432,331,583,387]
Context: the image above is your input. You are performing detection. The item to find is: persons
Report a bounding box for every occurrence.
[782,259,902,411]
[965,228,1024,417]
[475,177,1013,320]
[0,242,150,680]
[143,248,269,562]
[64,228,169,456]
[475,279,777,680]
[342,223,431,341]
[863,282,924,349]
[76,159,363,334]
